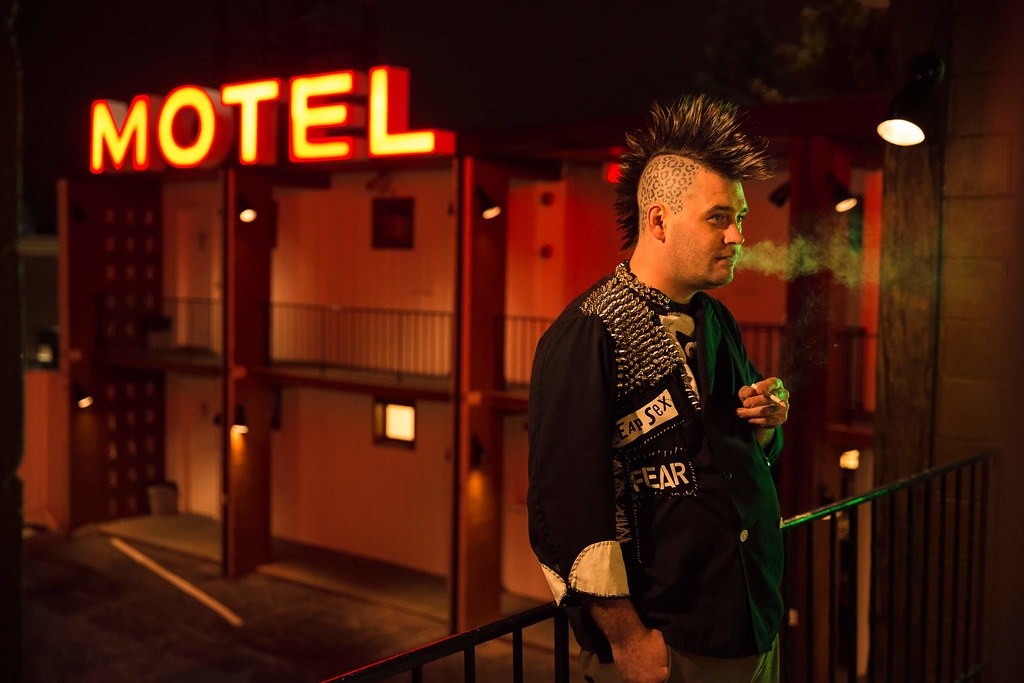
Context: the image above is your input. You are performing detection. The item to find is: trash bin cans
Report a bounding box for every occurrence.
[144,481,179,516]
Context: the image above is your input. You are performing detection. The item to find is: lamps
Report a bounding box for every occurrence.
[238,195,258,223]
[872,51,946,148]
[34,342,56,367]
[72,384,94,410]
[231,405,250,436]
[476,185,502,221]
[827,171,862,216]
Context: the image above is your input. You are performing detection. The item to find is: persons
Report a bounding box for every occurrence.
[525,101,791,683]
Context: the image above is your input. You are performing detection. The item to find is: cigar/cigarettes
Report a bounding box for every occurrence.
[751,382,787,409]
